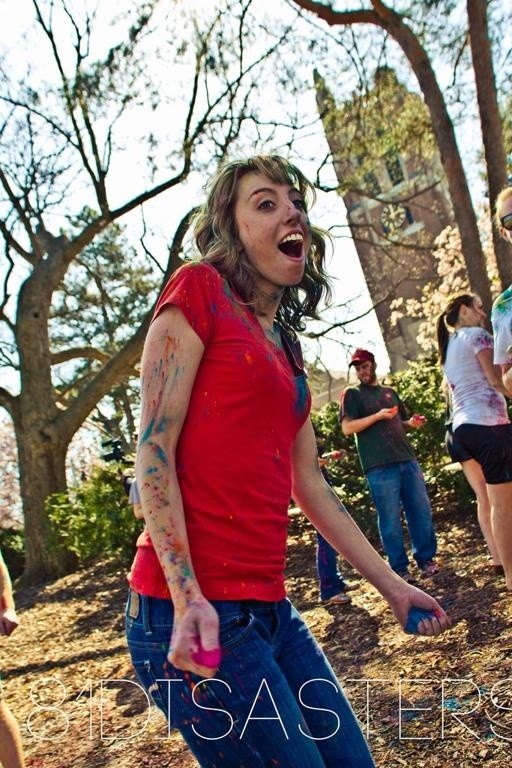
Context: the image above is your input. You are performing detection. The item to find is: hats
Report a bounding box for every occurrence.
[348,350,374,368]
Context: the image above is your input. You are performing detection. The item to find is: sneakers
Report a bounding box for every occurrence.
[323,593,351,604]
[341,583,360,591]
[420,561,439,577]
[398,573,416,584]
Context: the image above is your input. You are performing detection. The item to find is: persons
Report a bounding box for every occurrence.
[127,476,145,520]
[0,547,26,768]
[124,153,452,766]
[441,373,504,577]
[298,421,351,606]
[436,292,511,592]
[490,186,511,399]
[339,347,442,583]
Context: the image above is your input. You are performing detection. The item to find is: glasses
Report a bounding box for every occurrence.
[500,213,512,230]
